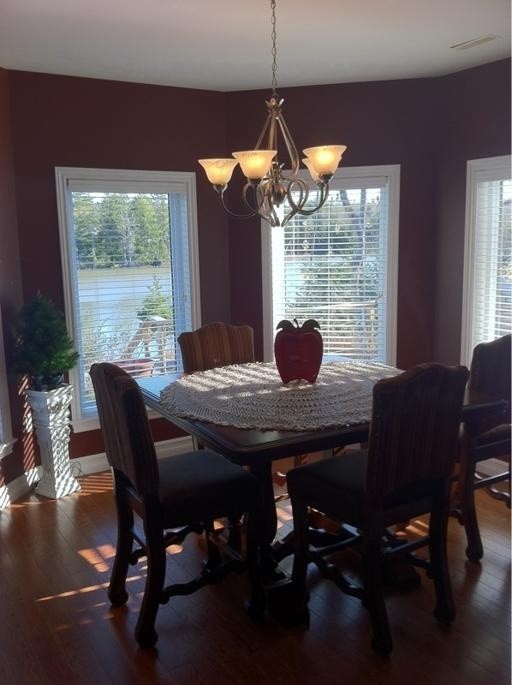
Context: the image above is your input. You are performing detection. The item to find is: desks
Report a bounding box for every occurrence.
[128,352,416,610]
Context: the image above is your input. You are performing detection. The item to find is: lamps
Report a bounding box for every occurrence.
[199,2,348,226]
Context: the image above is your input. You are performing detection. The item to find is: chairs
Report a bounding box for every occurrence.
[90,362,272,647]
[178,323,256,373]
[287,338,468,654]
[462,334,512,562]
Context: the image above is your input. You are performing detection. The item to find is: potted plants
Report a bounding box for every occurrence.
[10,292,81,499]
